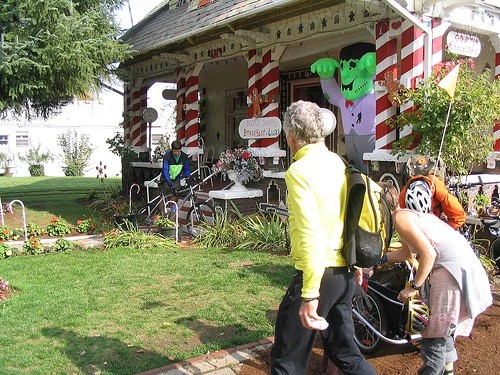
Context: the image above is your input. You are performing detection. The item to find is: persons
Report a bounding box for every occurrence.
[399,156,466,230]
[270,100,386,375]
[377,182,493,375]
[158,141,191,209]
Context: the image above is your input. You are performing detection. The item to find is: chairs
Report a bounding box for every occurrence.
[200,145,231,182]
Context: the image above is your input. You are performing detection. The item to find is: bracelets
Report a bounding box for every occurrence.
[304,298,320,302]
[410,280,420,290]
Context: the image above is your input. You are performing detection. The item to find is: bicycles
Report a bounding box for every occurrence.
[136,176,217,238]
[466,199,500,276]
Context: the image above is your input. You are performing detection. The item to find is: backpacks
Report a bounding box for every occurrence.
[339,154,389,270]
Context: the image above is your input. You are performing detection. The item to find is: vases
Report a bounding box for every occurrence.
[159,228,183,240]
[228,169,249,190]
[115,213,142,231]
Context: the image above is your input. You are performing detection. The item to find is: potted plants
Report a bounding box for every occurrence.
[16,142,55,176]
[472,193,490,218]
[459,193,468,213]
[57,130,92,176]
[1,151,16,176]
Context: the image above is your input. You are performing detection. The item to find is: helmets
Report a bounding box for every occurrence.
[399,296,429,333]
[404,187,432,213]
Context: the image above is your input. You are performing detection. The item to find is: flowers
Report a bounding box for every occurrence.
[111,200,144,216]
[153,215,181,230]
[211,148,264,185]
